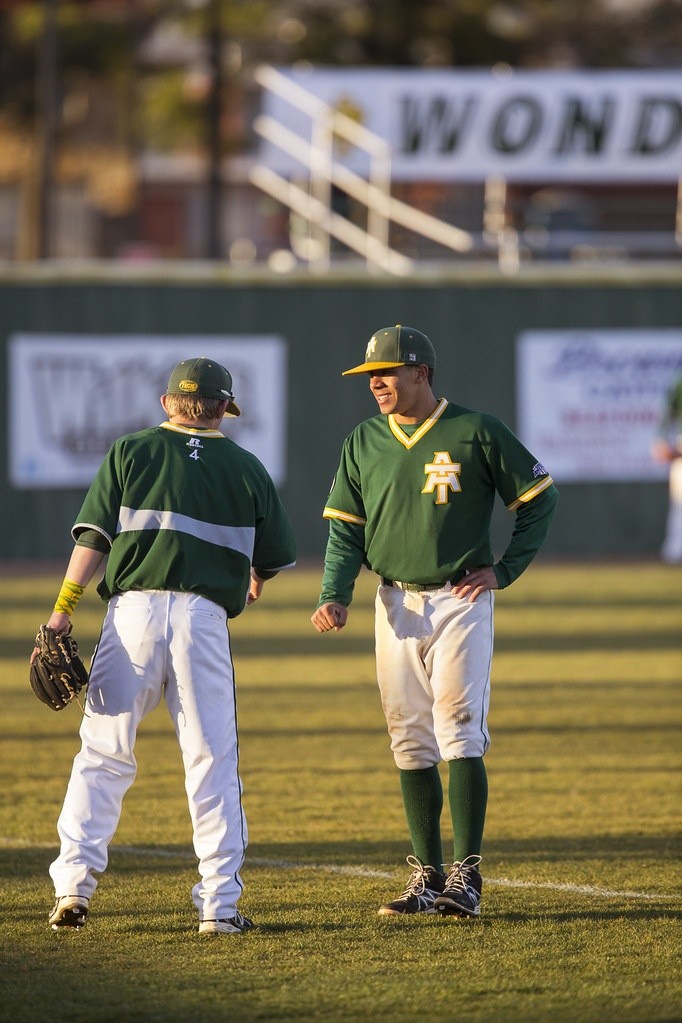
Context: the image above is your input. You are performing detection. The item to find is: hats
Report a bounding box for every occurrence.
[167,357,240,418]
[341,325,436,377]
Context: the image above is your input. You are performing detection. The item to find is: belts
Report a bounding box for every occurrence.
[383,577,447,592]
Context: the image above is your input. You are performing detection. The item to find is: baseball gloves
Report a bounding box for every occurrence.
[29,624,89,711]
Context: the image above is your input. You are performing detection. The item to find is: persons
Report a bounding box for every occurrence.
[309,323,559,916]
[650,374,682,568]
[30,355,296,938]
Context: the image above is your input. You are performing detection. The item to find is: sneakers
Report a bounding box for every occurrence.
[377,855,447,916]
[198,910,254,937]
[435,855,482,915]
[48,895,89,933]
